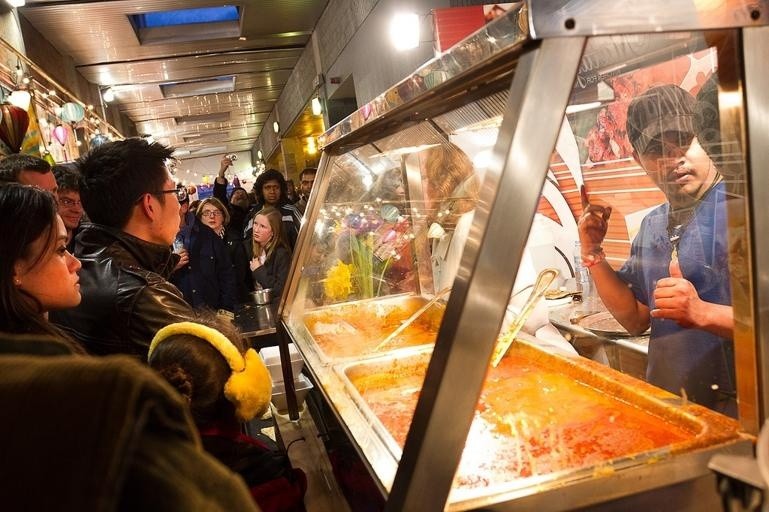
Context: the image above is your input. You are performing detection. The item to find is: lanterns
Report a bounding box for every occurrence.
[52,126,67,146]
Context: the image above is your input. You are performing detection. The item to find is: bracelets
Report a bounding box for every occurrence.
[580,246,606,265]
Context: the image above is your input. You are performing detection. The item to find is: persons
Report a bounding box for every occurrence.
[396,139,550,340]
[1,136,323,511]
[577,84,736,418]
[690,68,724,178]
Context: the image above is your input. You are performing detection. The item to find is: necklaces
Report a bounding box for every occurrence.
[665,170,721,278]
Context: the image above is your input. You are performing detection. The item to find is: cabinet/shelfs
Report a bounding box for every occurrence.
[274,0,769,511]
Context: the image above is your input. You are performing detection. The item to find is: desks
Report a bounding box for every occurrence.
[229,301,283,349]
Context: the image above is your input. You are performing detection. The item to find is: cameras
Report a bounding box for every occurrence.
[226,153,238,163]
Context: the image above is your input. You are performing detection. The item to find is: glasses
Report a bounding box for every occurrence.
[60,198,83,206]
[135,184,190,206]
[201,209,221,216]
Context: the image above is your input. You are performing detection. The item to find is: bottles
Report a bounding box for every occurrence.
[574,241,590,285]
[173,235,184,254]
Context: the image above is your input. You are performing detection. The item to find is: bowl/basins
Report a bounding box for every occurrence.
[249,288,273,305]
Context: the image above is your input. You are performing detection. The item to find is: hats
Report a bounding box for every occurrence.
[624,81,701,157]
